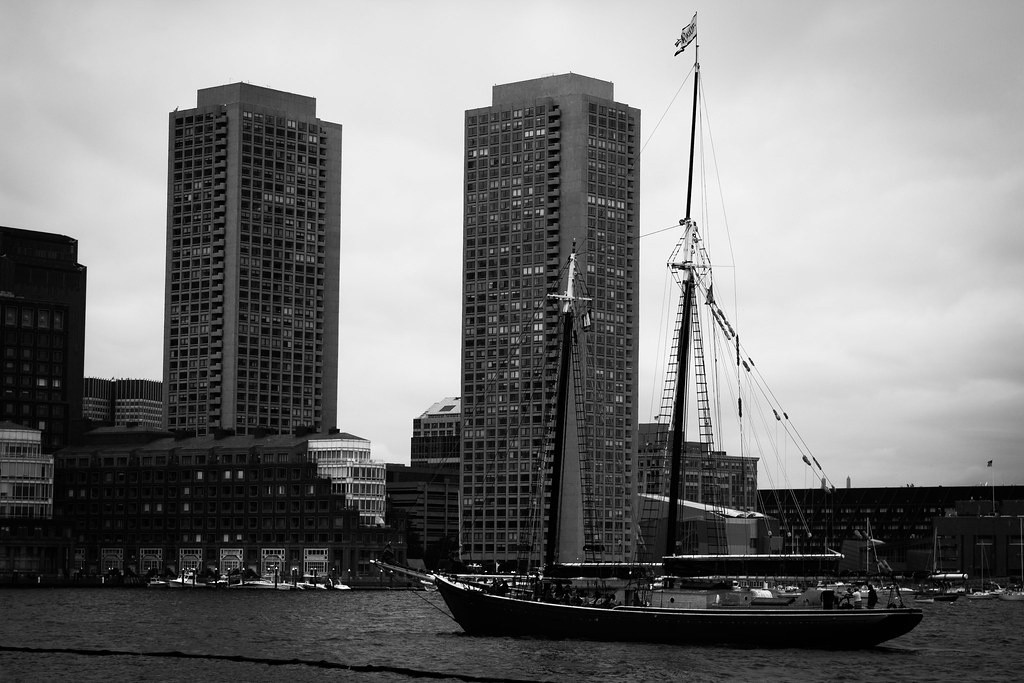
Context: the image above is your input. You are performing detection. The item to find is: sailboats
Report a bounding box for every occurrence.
[369,10,925,651]
[748,517,1024,607]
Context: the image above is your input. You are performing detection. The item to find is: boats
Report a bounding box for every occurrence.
[150,571,351,591]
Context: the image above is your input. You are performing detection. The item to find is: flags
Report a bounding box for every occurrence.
[987,460,992,467]
[673,13,699,57]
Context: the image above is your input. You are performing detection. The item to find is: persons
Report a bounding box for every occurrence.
[841,587,862,608]
[491,578,508,597]
[543,590,619,608]
[867,584,877,609]
